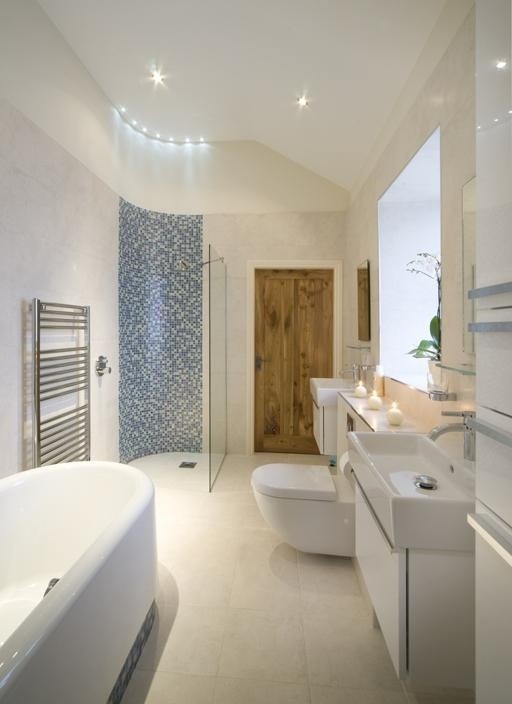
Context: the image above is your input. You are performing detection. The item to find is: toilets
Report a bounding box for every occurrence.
[251,463,355,558]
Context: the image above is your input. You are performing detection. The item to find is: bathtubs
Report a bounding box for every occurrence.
[0,460,157,704]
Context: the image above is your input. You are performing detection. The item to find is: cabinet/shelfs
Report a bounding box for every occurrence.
[464,0,512,703]
[309,378,425,482]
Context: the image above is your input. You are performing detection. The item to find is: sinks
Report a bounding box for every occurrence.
[347,431,476,551]
[310,377,353,407]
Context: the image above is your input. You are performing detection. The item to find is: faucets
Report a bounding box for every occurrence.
[429,410,476,461]
[338,364,360,383]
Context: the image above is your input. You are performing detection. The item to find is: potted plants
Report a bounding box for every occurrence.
[406,251,442,386]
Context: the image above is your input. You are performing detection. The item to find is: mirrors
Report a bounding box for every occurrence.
[356,259,373,343]
